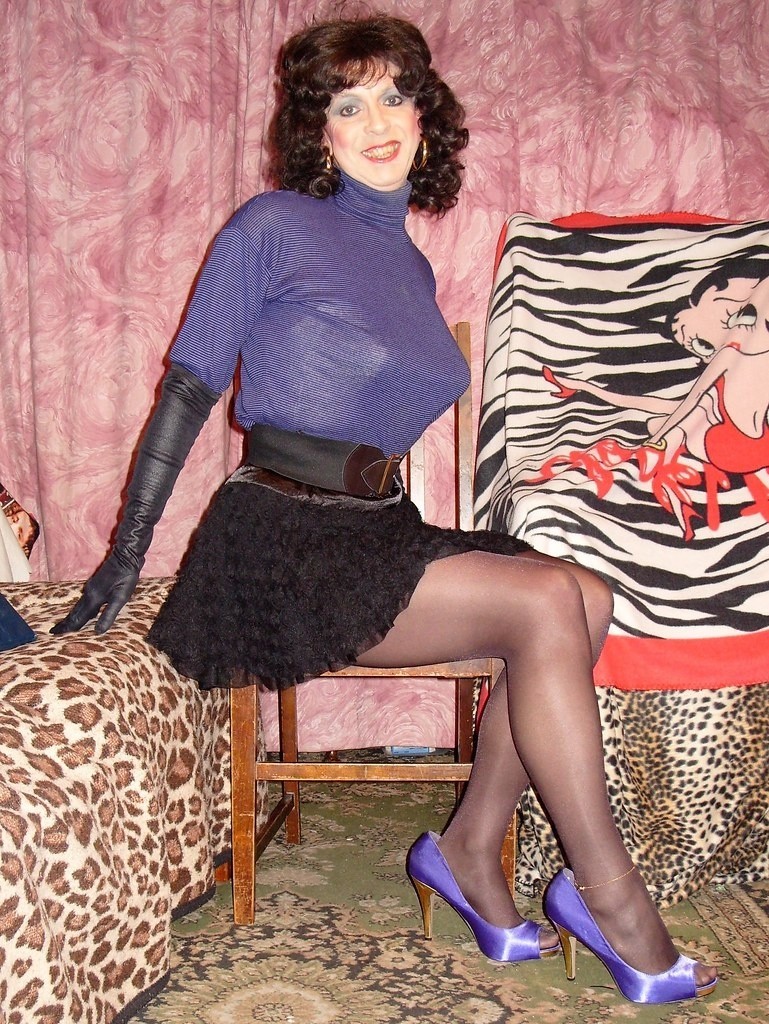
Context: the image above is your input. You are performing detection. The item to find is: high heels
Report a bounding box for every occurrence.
[541,868,719,1005]
[405,830,561,962]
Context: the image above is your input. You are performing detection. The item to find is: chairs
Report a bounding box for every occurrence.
[224,321,518,926]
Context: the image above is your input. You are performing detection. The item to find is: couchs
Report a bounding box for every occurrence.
[1,483,273,1022]
[478,210,769,908]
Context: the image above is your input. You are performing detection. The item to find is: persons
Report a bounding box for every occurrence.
[49,16,720,1005]
[7,510,38,551]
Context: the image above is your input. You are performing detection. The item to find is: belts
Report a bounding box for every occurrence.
[242,425,404,498]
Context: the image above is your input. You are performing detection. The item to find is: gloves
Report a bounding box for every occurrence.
[50,363,222,636]
